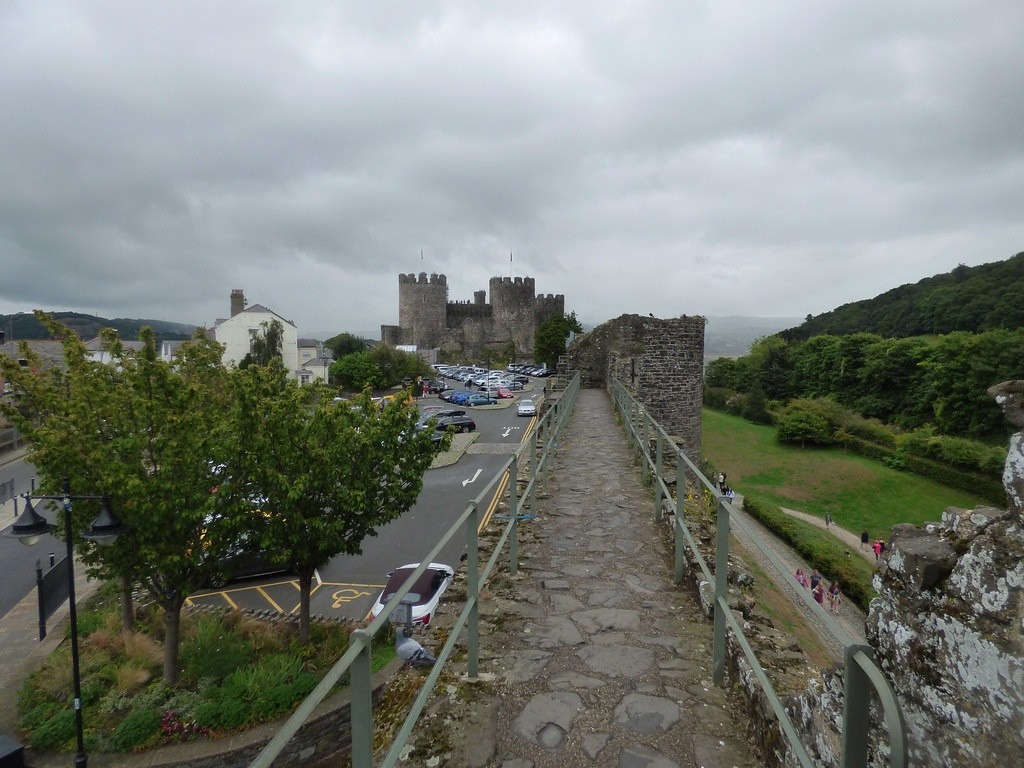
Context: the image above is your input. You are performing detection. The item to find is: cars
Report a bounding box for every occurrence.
[332,363,556,444]
[368,562,455,630]
[517,399,537,416]
[189,456,302,587]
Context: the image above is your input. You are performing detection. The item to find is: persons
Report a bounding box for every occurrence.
[423,384,449,398]
[872,537,885,559]
[825,512,831,531]
[464,379,472,390]
[713,470,734,505]
[795,569,841,616]
[860,529,870,552]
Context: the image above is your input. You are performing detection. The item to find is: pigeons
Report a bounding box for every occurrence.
[395,626,437,669]
[649,313,654,318]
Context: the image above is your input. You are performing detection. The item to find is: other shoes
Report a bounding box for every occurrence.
[859,547,862,548]
[831,609,834,611]
[865,550,869,552]
[836,612,840,615]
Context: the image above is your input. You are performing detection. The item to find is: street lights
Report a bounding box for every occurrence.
[11,490,124,768]
